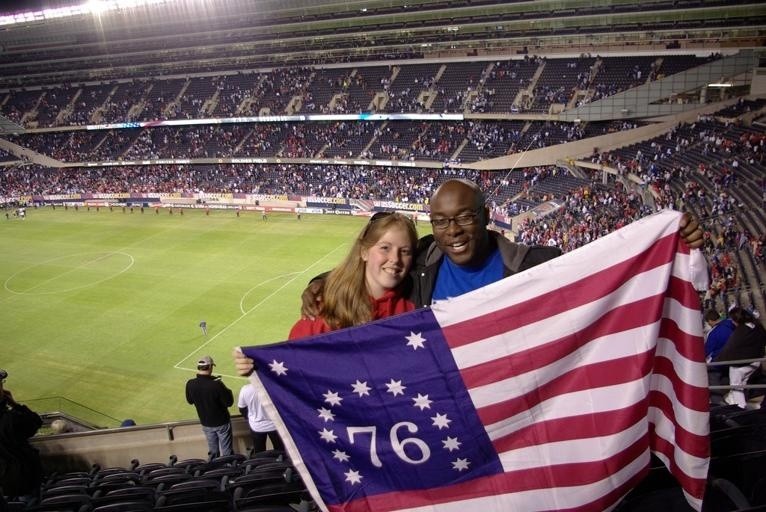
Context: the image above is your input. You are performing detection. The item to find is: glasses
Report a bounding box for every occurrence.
[430,206,482,229]
[362,211,391,238]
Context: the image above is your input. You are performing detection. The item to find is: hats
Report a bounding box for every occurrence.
[197,355,216,367]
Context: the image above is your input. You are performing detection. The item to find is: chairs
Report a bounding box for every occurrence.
[2,447,319,510]
[630,255,764,512]
[0,55,766,254]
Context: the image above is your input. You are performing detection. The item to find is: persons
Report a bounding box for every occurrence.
[122,419,136,427]
[50,420,74,433]
[299,178,704,320]
[705,305,766,407]
[1,37,763,307]
[186,355,234,459]
[2,372,42,495]
[234,213,415,375]
[239,384,284,451]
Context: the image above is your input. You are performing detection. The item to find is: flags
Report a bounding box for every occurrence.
[234,206,713,510]
